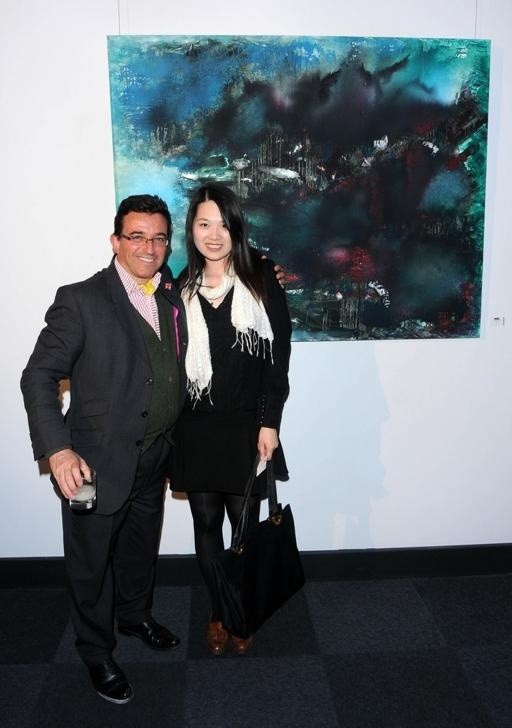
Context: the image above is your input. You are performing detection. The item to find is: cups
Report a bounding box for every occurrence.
[69,468,97,510]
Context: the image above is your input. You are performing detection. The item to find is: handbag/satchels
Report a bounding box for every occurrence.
[211,504,306,640]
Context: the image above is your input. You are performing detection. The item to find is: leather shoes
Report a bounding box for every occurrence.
[89,657,134,704]
[118,618,181,651]
[206,621,253,655]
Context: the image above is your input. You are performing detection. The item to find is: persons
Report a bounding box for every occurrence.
[20,195,287,704]
[177,185,292,656]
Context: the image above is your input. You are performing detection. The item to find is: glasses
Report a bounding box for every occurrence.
[120,234,169,247]
